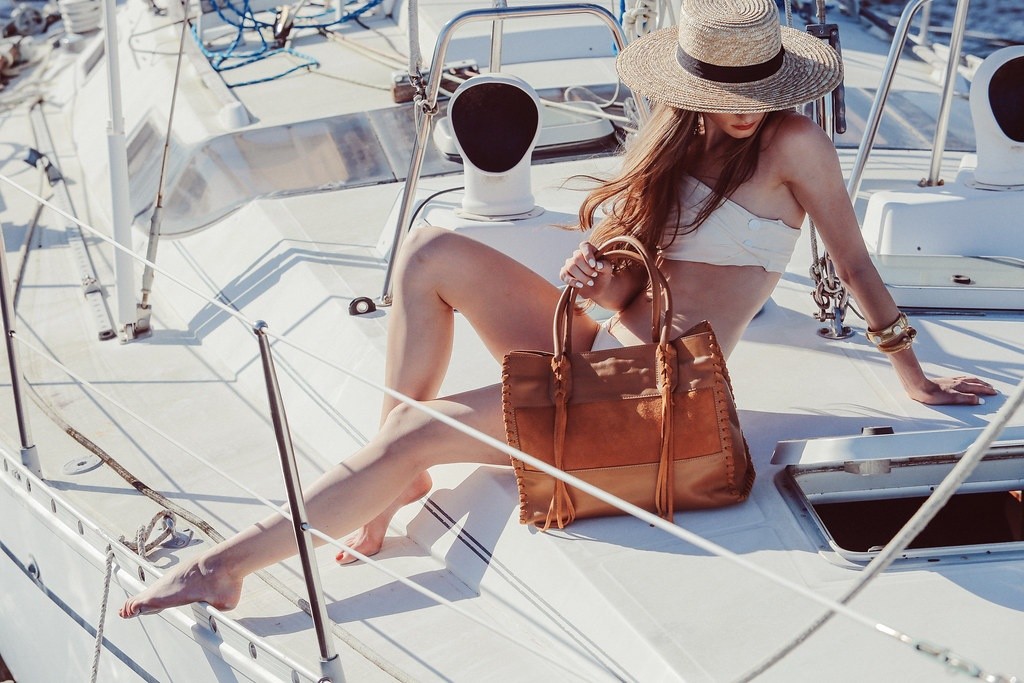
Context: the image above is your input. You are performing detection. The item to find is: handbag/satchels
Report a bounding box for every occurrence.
[501,235,756,531]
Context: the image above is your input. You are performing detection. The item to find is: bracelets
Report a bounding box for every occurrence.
[865,310,908,346]
[877,326,916,354]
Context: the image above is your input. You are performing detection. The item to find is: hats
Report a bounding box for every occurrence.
[614,0,844,114]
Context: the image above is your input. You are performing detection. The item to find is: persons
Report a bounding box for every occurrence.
[119,0,997,618]
